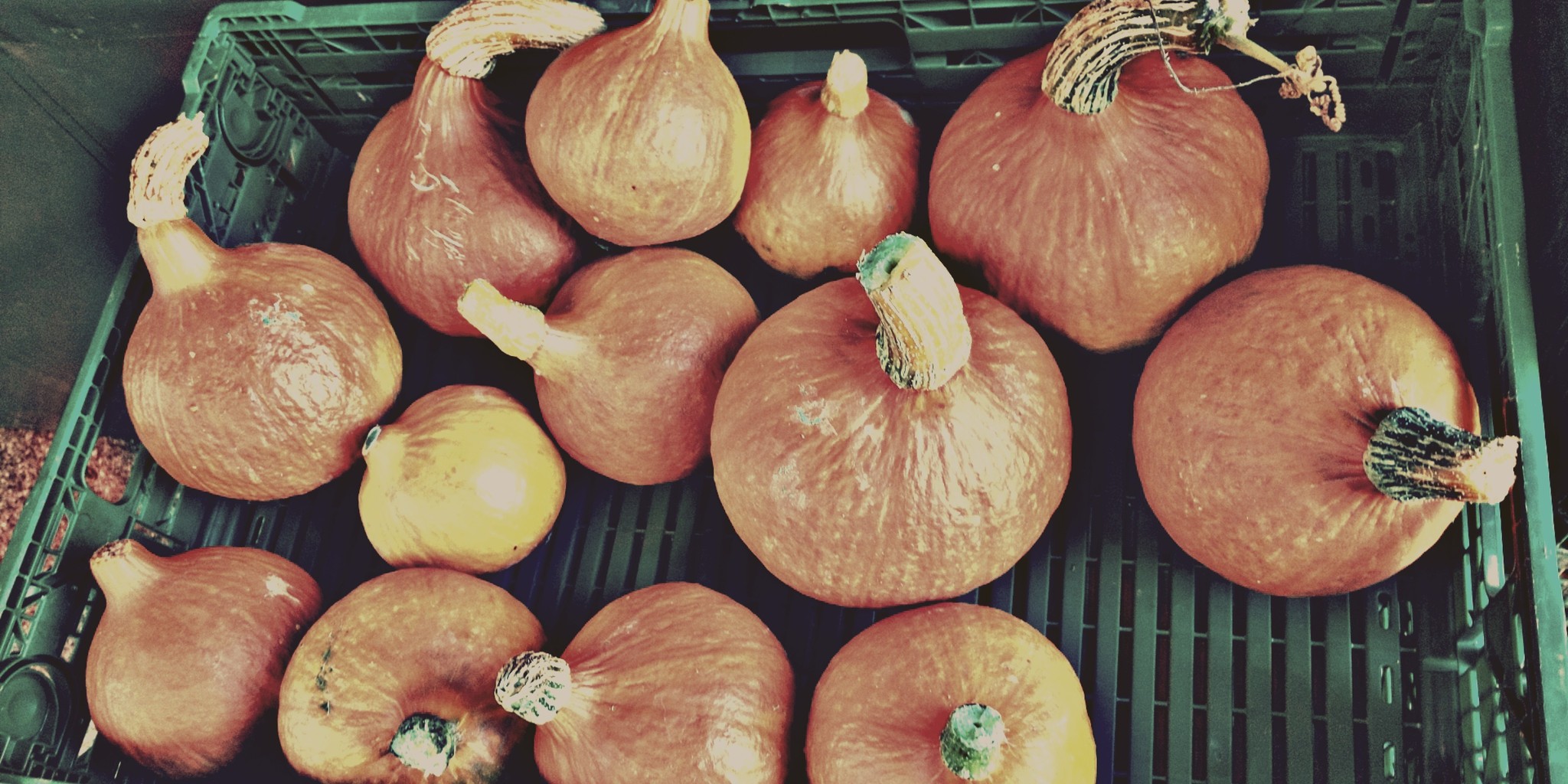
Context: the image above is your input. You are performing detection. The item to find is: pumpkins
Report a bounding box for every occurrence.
[84,0,1522,784]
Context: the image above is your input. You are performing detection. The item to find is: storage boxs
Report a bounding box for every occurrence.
[1,4,1567,781]
